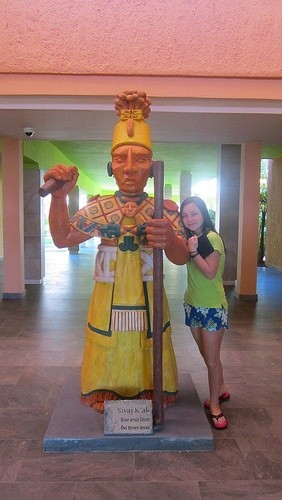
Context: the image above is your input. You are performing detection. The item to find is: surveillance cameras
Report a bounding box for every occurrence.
[23,127,34,137]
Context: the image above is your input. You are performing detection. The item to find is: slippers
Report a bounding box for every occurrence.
[204,392,230,409]
[210,410,228,429]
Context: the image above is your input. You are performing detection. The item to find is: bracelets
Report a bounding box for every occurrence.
[189,251,199,258]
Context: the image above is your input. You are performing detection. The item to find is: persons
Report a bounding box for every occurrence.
[43,121,189,414]
[180,196,231,428]
